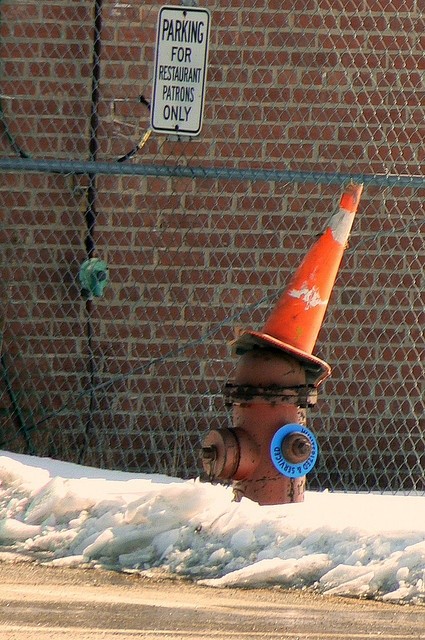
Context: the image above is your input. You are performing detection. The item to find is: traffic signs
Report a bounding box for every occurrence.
[149,5,212,136]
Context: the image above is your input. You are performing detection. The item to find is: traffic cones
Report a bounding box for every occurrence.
[238,181,364,388]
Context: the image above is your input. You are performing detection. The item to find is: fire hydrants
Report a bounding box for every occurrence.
[203,338,317,505]
